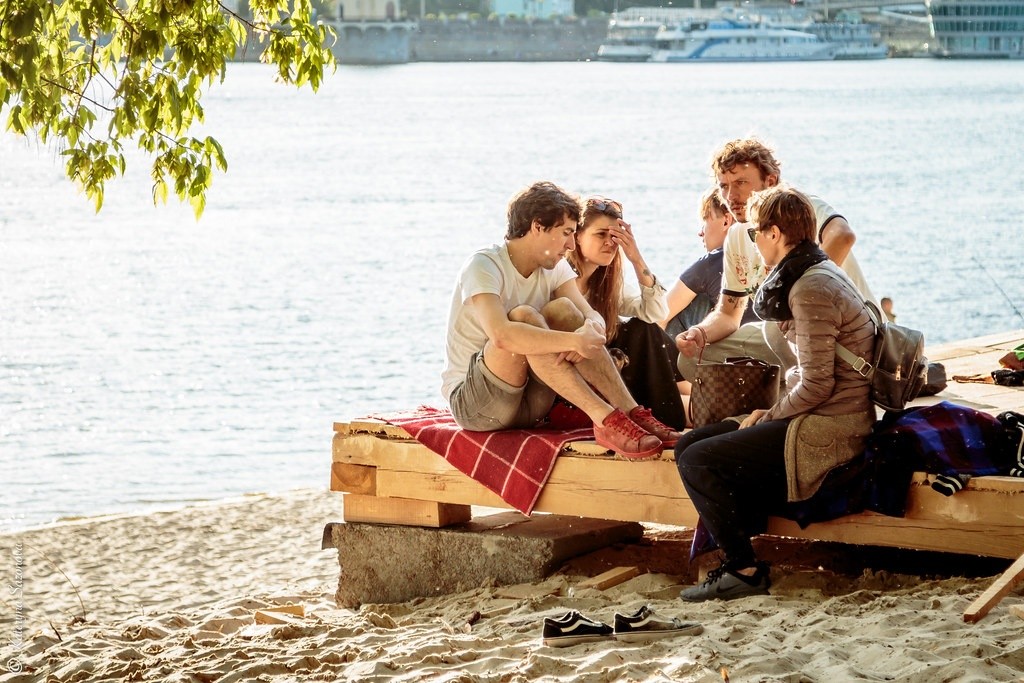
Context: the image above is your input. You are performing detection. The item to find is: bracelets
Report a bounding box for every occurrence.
[694,322,711,348]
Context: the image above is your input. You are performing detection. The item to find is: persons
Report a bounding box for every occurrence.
[441,182,683,458]
[564,197,693,433]
[674,187,877,602]
[655,139,897,404]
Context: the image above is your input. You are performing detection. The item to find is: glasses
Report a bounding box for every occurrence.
[747,227,759,243]
[586,199,623,220]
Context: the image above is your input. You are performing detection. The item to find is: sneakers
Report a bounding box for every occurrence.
[613,603,703,642]
[543,610,613,647]
[593,405,684,458]
[679,554,771,602]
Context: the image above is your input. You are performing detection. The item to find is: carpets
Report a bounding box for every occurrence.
[356,407,596,518]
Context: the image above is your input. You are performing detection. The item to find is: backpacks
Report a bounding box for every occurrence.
[800,269,927,412]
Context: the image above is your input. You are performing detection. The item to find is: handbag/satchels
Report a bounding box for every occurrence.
[688,356,780,428]
[544,385,611,430]
